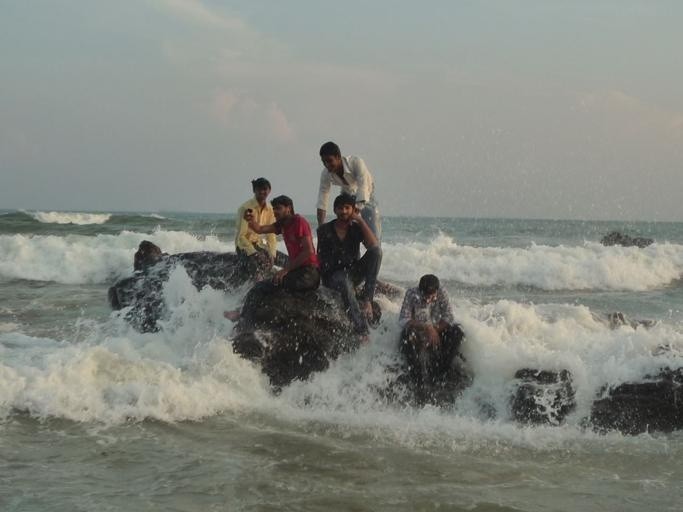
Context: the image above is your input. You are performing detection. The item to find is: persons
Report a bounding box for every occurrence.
[222,141,382,345]
[398,274,463,377]
